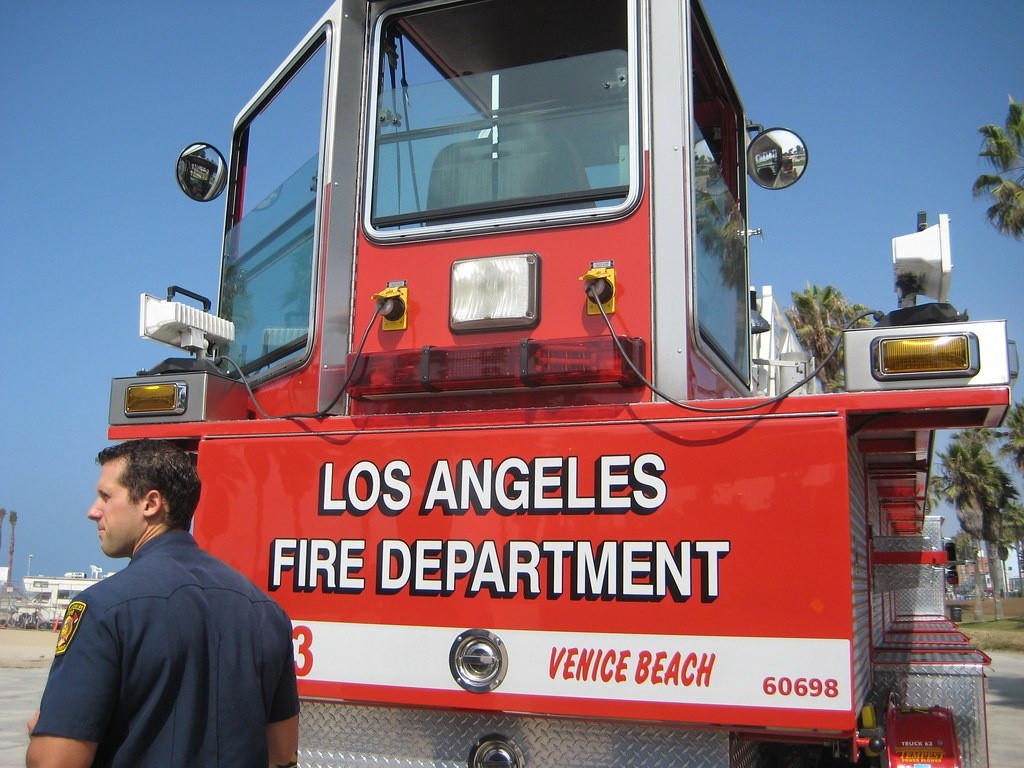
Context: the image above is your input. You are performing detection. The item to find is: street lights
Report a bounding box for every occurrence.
[25,553,36,575]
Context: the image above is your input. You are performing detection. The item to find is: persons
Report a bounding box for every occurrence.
[26,438,300,768]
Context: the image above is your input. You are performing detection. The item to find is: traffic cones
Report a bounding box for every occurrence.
[51,619,61,633]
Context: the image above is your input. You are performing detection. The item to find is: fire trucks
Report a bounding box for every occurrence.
[108,0,1011,767]
